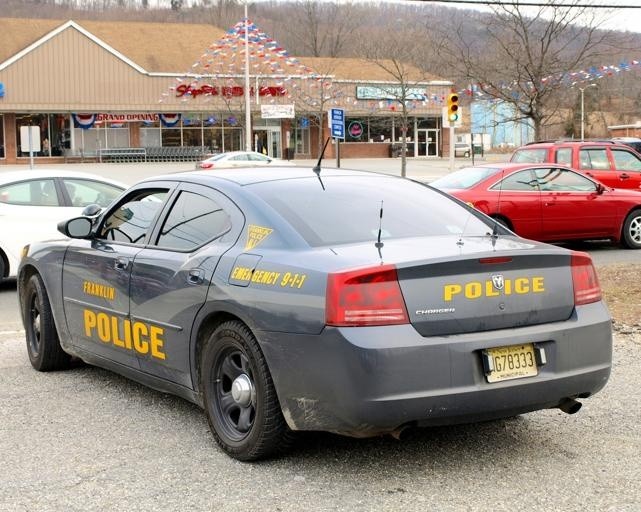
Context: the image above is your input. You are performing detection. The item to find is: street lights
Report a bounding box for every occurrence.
[572,81,596,141]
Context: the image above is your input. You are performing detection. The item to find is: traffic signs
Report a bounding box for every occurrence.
[330,108,345,140]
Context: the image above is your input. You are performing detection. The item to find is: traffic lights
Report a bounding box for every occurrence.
[447,93,458,122]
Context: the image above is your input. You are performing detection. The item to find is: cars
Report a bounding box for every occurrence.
[195,150,295,170]
[454,143,470,157]
[509,139,640,191]
[16,164,613,465]
[0,168,163,284]
[428,162,641,250]
